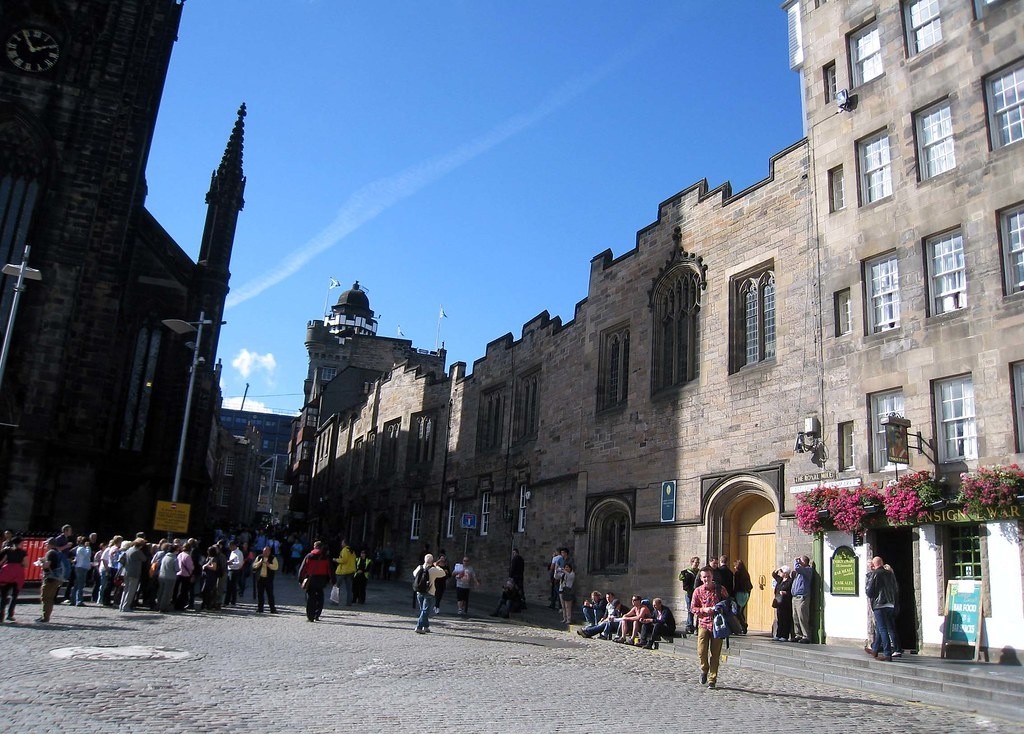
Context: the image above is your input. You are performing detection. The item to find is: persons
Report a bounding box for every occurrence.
[412,547,904,662]
[693,567,729,687]
[890,425,907,459]
[0,516,394,626]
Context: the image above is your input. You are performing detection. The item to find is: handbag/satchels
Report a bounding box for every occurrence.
[207,557,217,571]
[713,583,742,649]
[190,575,197,583]
[114,576,124,586]
[300,578,311,590]
[329,585,340,603]
[149,561,160,578]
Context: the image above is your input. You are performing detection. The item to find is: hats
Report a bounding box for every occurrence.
[611,599,620,608]
[780,566,791,574]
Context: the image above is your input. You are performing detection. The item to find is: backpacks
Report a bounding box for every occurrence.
[641,598,654,612]
[45,549,71,580]
[412,565,435,593]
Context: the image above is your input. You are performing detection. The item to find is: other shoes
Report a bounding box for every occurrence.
[791,637,810,644]
[616,637,634,645]
[636,642,653,649]
[686,627,698,636]
[597,636,607,640]
[577,627,589,638]
[743,623,748,634]
[891,652,902,657]
[414,626,430,634]
[774,637,788,641]
[708,683,715,688]
[432,606,440,614]
[864,648,892,662]
[458,608,464,615]
[699,673,707,684]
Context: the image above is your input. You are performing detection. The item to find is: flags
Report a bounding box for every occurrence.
[329,279,341,289]
[398,327,404,337]
[440,309,447,318]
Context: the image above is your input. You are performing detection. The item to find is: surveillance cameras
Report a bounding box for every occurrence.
[199,357,205,365]
